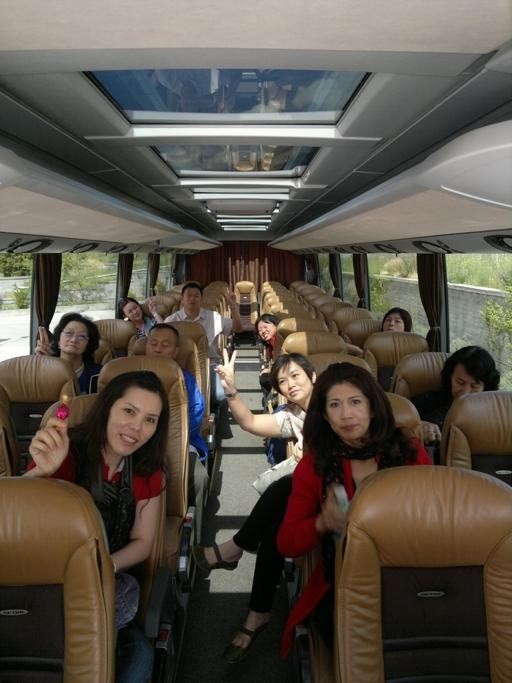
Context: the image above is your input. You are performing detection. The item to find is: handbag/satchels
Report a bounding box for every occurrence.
[251,455,299,496]
[96,468,138,554]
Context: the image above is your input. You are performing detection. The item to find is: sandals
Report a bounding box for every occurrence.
[224,617,268,662]
[192,542,239,571]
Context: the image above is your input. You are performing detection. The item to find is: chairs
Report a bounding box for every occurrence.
[0,281,231,677]
[259,282,512,677]
[231,282,258,345]
